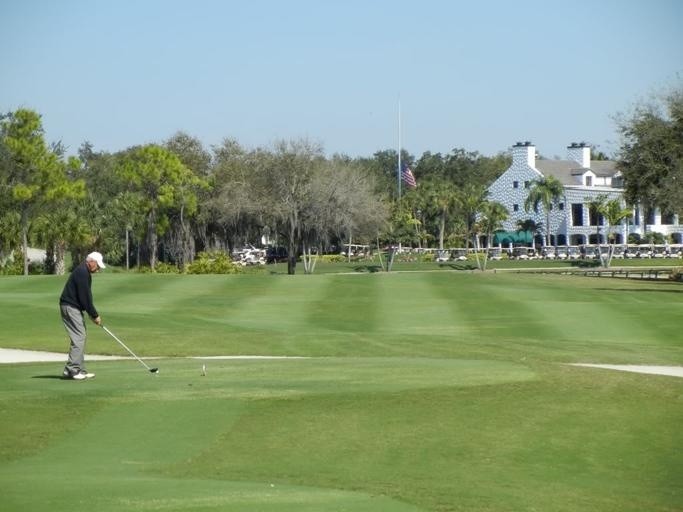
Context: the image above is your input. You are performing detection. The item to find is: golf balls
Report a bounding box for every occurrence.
[156,370,159,373]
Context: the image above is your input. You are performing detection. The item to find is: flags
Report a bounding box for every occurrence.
[401,158,417,187]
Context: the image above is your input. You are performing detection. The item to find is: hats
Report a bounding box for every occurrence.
[88,252,105,269]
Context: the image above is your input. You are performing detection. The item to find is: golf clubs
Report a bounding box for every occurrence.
[100,324,158,373]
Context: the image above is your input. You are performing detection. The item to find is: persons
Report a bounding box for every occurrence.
[59,251,106,380]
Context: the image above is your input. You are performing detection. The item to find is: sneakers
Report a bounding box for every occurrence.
[63,371,95,379]
